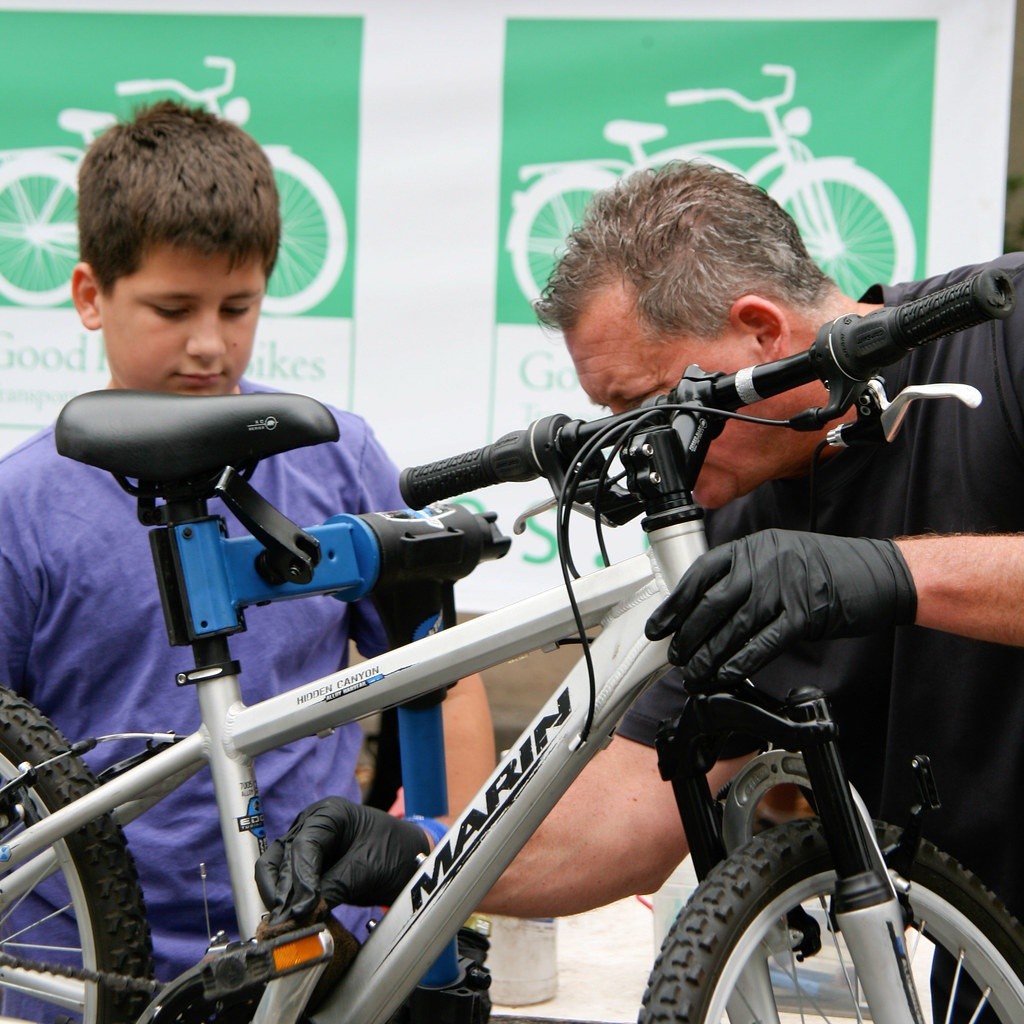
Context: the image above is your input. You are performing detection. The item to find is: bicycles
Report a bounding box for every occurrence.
[1,265,1022,1024]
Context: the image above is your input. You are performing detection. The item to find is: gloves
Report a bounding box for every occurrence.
[645,529,919,701]
[254,795,430,925]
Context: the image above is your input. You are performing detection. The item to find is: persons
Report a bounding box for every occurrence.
[0,102,492,1024]
[252,164,1022,1022]
